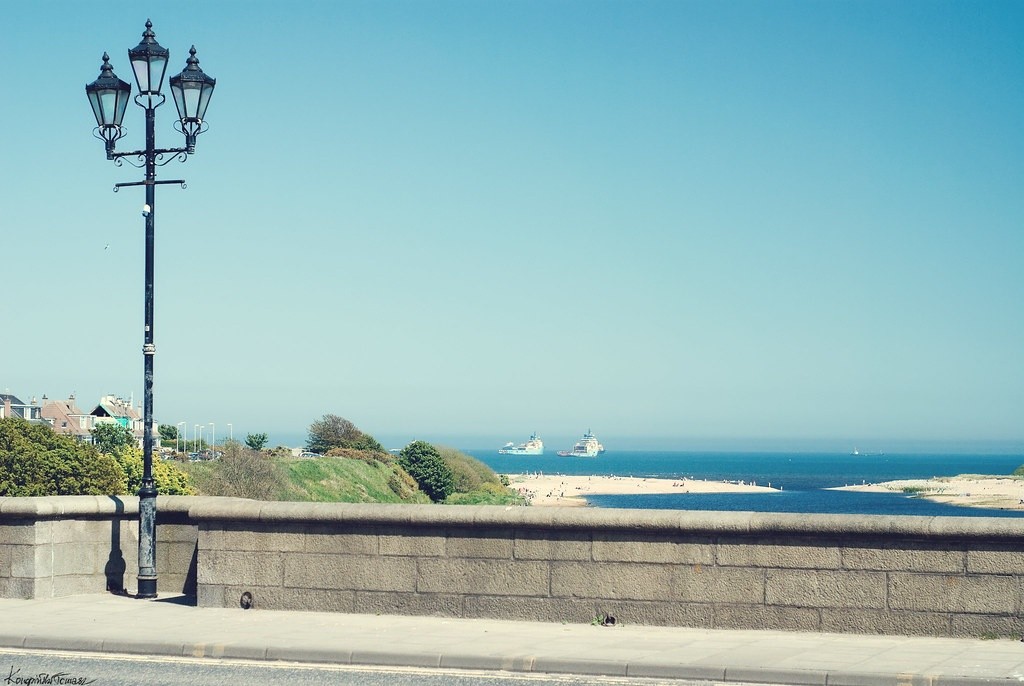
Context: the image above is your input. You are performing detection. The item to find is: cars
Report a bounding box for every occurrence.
[299,451,320,458]
[187,449,226,461]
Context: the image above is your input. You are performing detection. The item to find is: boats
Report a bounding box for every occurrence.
[850,446,859,455]
[498,431,544,456]
[599,442,605,454]
[556,450,574,457]
[572,428,599,458]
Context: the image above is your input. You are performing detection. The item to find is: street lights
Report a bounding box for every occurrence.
[209,423,216,459]
[176,422,205,455]
[85,16,216,599]
[227,423,232,444]
